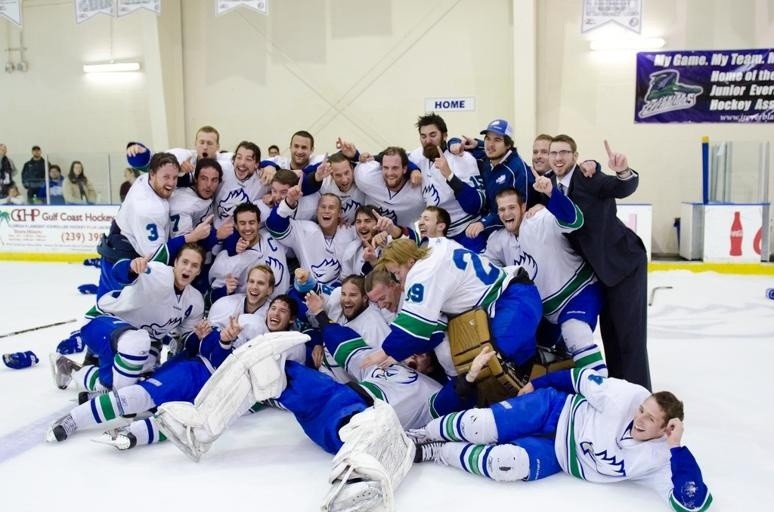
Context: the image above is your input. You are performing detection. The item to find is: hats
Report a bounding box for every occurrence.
[480,120,514,139]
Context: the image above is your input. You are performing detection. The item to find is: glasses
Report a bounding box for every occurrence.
[550,151,573,158]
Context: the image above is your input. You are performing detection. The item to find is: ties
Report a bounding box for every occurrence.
[557,183,564,195]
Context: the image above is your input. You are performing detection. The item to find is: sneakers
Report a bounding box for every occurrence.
[414,442,444,463]
[56,353,72,389]
[52,414,76,442]
[159,408,206,455]
[103,428,136,450]
[79,391,101,405]
[322,481,383,512]
[404,426,434,444]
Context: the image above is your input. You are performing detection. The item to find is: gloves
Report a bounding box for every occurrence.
[84,259,101,268]
[57,330,84,354]
[3,351,39,368]
[78,284,99,295]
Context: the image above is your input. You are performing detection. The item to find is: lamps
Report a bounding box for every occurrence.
[81,57,143,73]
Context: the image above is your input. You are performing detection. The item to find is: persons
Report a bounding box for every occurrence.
[38,165,66,204]
[48,113,713,512]
[22,146,52,206]
[0,144,17,197]
[0,186,28,205]
